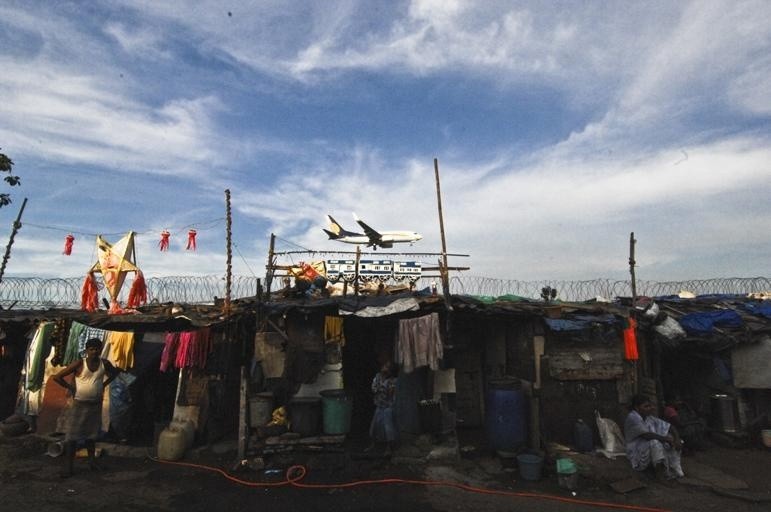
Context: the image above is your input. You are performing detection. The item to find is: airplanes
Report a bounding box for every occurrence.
[322,212,425,252]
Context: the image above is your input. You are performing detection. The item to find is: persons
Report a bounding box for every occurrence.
[53,337,122,479]
[364,360,400,459]
[664,389,715,456]
[623,393,692,487]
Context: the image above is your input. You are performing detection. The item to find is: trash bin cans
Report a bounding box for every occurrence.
[708,393,741,432]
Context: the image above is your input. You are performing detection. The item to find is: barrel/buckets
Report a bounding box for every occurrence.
[249,398,275,429]
[157,418,193,461]
[711,394,740,433]
[416,395,444,436]
[761,429,771,448]
[517,454,543,482]
[488,379,527,454]
